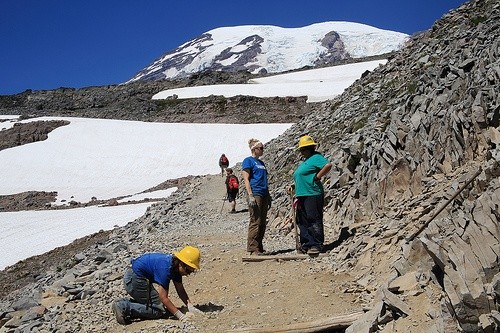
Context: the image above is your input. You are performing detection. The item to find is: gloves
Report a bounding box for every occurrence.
[247,194,257,207]
[174,303,202,321]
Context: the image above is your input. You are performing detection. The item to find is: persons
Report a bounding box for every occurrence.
[242,138,272,256]
[219,154,229,177]
[225,168,240,213]
[293,136,332,254]
[112,246,204,326]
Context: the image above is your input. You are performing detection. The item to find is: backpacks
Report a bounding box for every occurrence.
[228,175,239,191]
[221,157,227,164]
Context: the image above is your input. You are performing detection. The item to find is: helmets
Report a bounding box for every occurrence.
[174,246,201,271]
[297,135,317,150]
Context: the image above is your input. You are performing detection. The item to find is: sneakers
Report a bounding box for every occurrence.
[113,298,129,326]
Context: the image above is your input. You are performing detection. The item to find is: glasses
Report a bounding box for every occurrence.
[254,146,263,150]
[179,261,193,275]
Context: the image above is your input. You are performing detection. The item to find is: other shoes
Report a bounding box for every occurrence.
[228,210,236,213]
